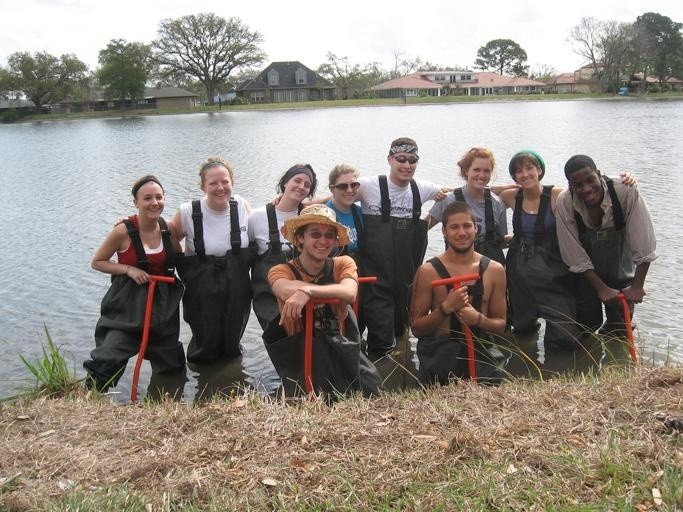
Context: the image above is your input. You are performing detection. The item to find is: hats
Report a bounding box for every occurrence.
[284,204,352,247]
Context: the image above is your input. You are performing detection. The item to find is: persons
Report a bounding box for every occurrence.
[82,176,185,394]
[113,158,254,391]
[267,204,389,404]
[251,137,654,359]
[412,202,528,387]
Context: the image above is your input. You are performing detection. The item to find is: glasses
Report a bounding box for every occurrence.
[311,232,335,239]
[395,155,417,163]
[332,182,360,190]
